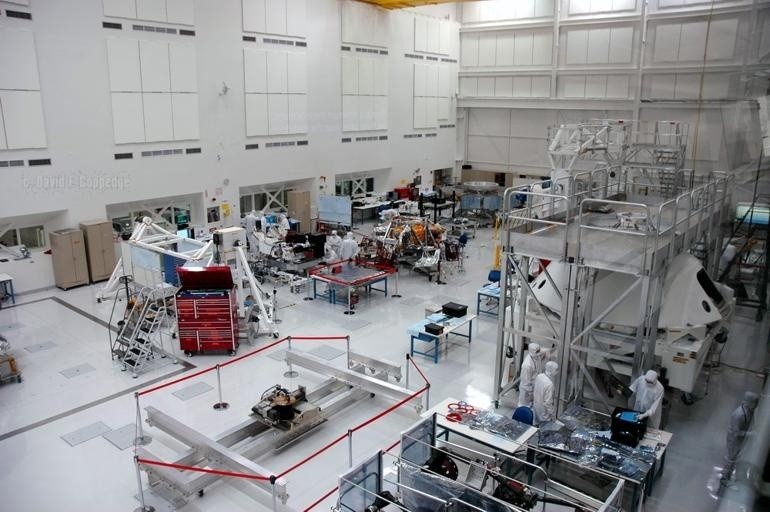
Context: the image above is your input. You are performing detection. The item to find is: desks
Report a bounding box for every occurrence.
[0,271,16,307]
[308,261,399,313]
[408,275,517,364]
[416,383,676,507]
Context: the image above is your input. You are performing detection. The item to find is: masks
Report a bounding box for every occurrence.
[645,381,655,387]
[536,351,542,357]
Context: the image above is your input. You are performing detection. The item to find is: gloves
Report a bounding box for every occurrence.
[617,389,621,395]
[636,413,648,419]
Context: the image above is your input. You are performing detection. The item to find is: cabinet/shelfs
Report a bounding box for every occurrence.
[286,190,312,235]
[48,217,120,289]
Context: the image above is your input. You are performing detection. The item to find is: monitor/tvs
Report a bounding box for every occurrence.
[734,202,770,226]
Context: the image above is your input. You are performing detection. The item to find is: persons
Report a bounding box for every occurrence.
[338,231,359,262]
[279,213,291,232]
[318,243,339,293]
[616,370,665,430]
[325,229,343,256]
[530,359,559,443]
[517,342,550,409]
[242,209,259,256]
[719,390,761,493]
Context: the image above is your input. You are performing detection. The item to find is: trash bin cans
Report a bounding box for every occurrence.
[660,397,671,429]
